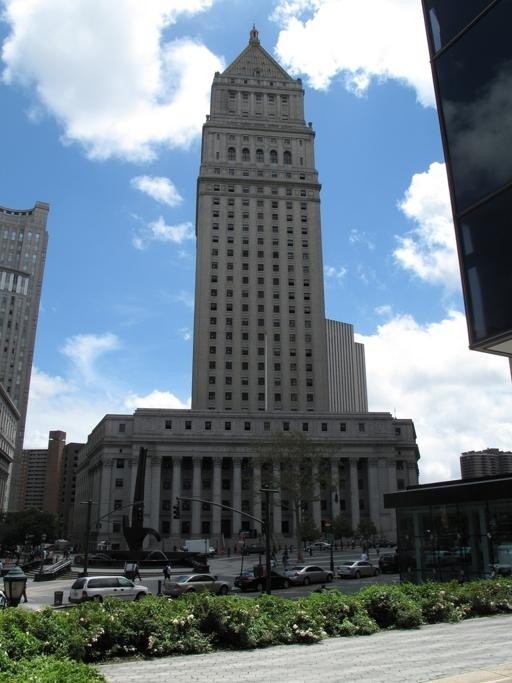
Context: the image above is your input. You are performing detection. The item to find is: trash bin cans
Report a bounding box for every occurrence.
[54,591,62,605]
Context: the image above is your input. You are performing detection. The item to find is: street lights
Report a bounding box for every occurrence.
[3,566,26,606]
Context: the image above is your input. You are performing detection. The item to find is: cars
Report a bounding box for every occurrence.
[163,574,232,596]
[305,542,332,552]
[285,566,333,586]
[423,546,511,572]
[379,553,414,572]
[234,566,290,590]
[337,561,381,579]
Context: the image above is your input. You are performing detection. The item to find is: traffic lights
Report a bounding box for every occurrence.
[172,505,180,520]
[135,505,144,521]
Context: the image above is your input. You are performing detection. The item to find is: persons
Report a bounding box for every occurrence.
[132,561,141,582]
[15,543,70,603]
[163,565,170,585]
[328,539,369,560]
[270,544,312,568]
[209,544,216,557]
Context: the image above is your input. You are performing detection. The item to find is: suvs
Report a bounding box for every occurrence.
[69,575,150,602]
